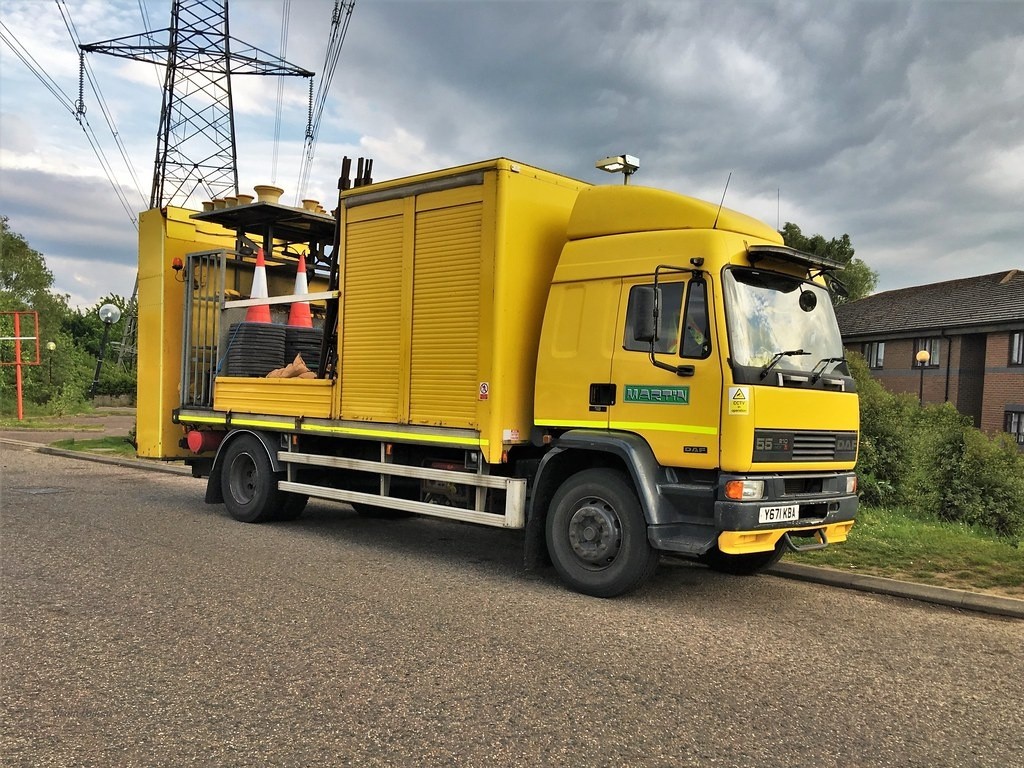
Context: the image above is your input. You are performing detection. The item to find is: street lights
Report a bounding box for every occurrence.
[88,303,122,399]
[915,350,931,407]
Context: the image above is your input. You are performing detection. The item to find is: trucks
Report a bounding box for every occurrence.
[135,154,868,597]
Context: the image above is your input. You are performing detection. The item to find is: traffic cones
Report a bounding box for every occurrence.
[225,246,325,376]
